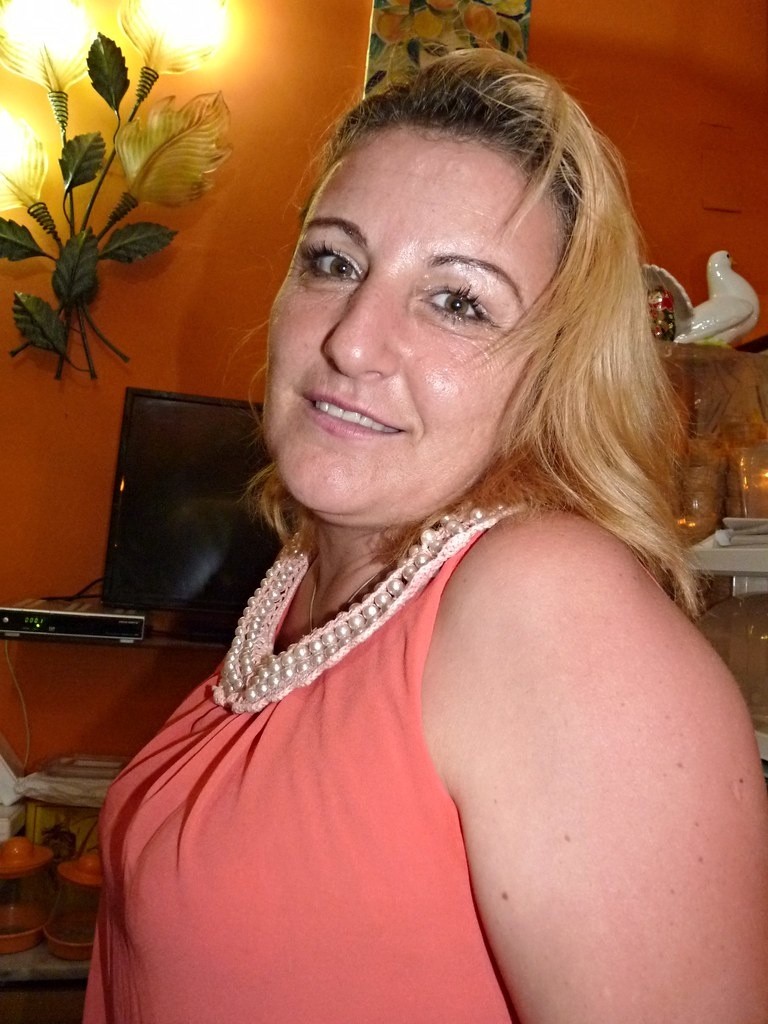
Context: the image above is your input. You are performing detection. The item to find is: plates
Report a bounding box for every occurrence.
[695,596,768,733]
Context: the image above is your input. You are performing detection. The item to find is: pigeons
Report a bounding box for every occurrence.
[674,250,760,350]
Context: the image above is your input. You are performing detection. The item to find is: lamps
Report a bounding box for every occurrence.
[1,0,242,380]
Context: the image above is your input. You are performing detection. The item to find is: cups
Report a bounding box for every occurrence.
[43,849,104,960]
[678,422,760,545]
[0,836,52,953]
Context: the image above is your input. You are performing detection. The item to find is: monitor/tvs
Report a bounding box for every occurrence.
[100,387,292,613]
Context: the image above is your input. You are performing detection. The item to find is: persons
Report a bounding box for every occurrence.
[80,50,768,1024]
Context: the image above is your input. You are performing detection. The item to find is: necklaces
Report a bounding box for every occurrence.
[308,568,380,632]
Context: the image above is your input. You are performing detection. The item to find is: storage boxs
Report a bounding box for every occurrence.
[26,799,101,862]
